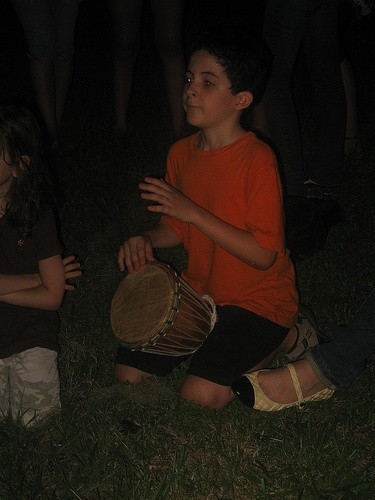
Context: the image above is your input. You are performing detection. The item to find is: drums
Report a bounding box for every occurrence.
[109,262,215,356]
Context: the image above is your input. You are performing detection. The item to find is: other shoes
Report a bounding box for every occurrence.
[232,362,338,413]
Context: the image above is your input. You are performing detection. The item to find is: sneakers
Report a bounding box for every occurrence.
[284,305,324,362]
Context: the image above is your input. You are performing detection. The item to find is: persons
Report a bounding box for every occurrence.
[115,31,321,412]
[265,0,347,185]
[0,102,82,431]
[13,0,80,139]
[249,0,362,158]
[102,0,185,140]
